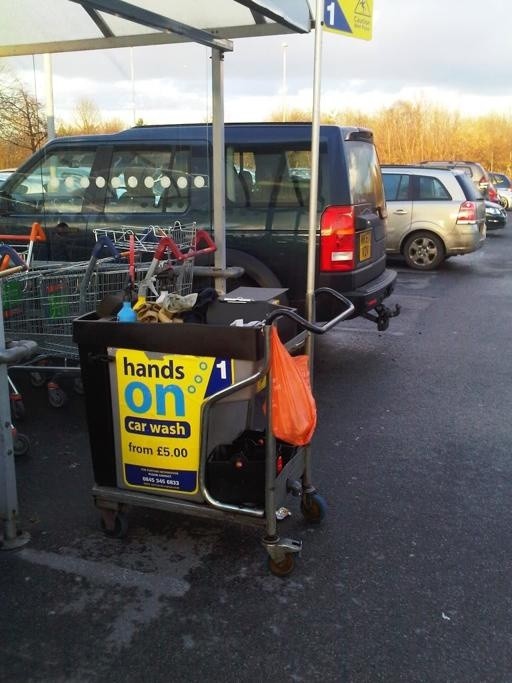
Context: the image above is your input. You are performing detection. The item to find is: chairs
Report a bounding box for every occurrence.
[108,163,253,213]
